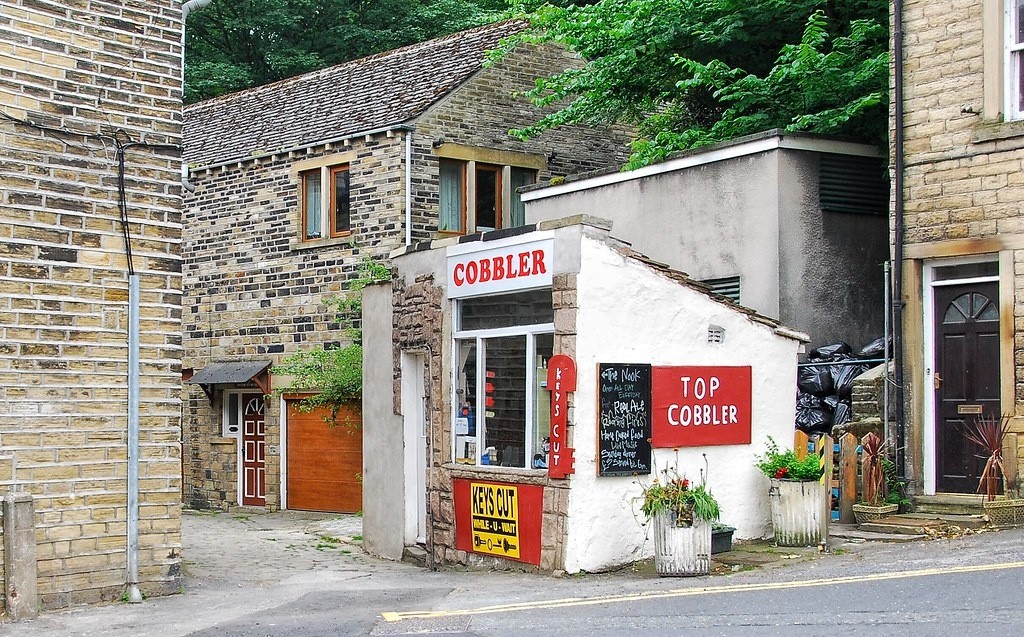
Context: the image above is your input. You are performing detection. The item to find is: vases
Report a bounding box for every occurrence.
[652,500,712,577]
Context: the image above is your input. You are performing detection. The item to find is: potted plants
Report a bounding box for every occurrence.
[753,435,828,547]
[852,436,900,527]
[955,412,1024,529]
[711,523,738,552]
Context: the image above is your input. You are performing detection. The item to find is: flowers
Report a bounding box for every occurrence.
[632,439,722,540]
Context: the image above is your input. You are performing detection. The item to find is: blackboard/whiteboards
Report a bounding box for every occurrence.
[597,362,652,477]
